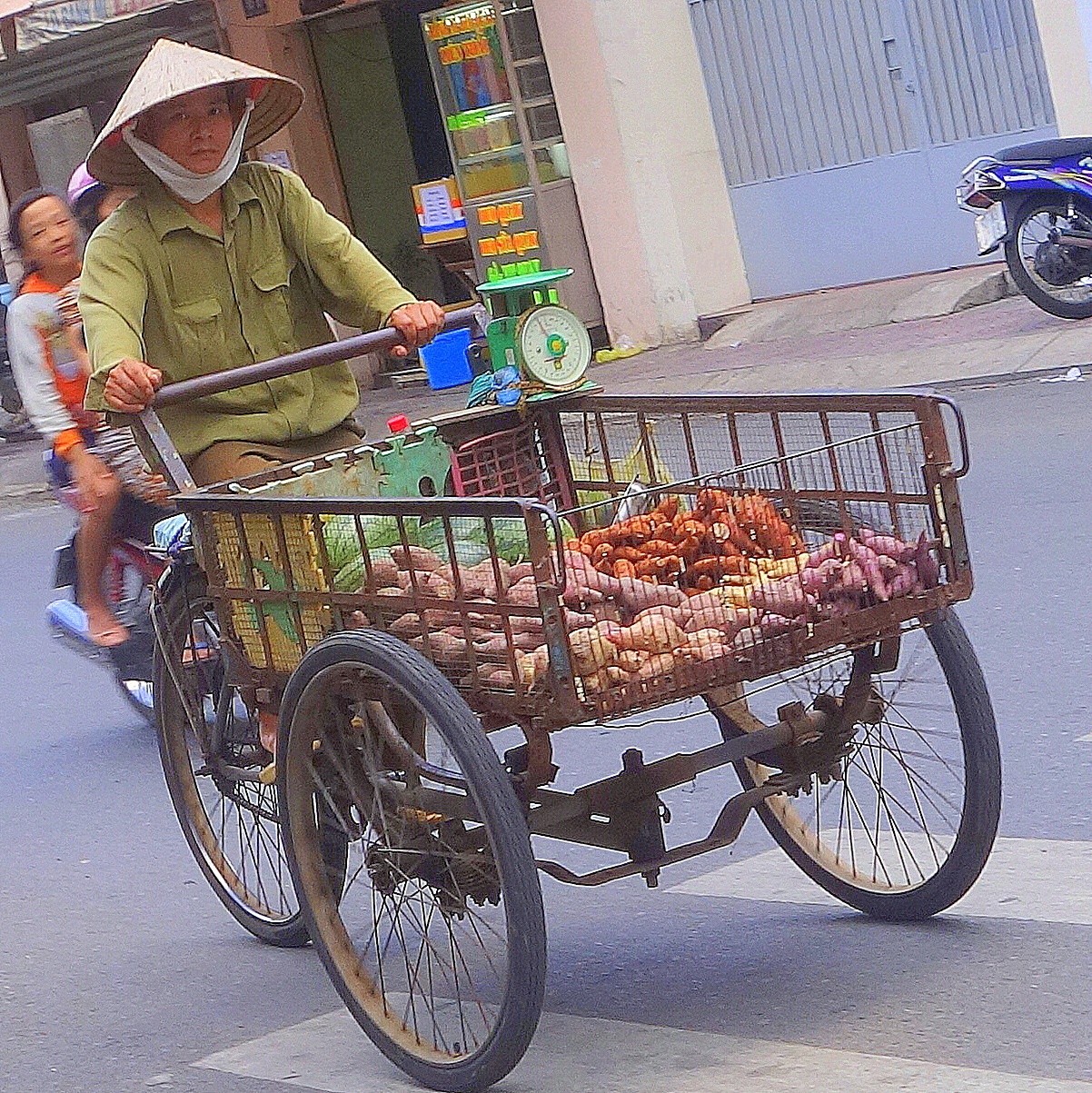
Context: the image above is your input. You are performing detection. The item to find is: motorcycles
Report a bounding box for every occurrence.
[954,133,1092,319]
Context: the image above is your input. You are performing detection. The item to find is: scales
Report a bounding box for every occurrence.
[475,268,591,386]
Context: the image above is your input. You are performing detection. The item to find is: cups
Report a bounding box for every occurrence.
[612,482,652,526]
[548,143,571,180]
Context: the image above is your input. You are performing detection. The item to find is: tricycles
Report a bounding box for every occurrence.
[126,304,1004,1093]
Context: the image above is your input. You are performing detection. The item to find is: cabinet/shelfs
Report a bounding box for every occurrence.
[419,0,611,349]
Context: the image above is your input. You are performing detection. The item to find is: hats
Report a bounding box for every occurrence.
[86,37,305,186]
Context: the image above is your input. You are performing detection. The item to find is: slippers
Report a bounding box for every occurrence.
[395,807,443,825]
[254,717,363,786]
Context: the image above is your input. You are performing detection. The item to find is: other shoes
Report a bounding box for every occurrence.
[82,606,129,645]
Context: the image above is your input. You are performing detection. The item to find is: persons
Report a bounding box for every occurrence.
[5,162,175,646]
[77,35,443,824]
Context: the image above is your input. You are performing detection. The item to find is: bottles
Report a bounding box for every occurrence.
[386,415,411,437]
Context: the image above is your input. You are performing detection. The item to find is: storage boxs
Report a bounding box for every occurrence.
[411,175,466,243]
[420,325,472,389]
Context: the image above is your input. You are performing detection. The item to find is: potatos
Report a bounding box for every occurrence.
[339,489,944,699]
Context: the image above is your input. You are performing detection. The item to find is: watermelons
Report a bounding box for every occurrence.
[314,508,580,598]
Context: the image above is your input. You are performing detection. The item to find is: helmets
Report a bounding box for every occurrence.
[67,161,104,214]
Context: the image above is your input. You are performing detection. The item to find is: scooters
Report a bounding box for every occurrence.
[34,443,264,747]
